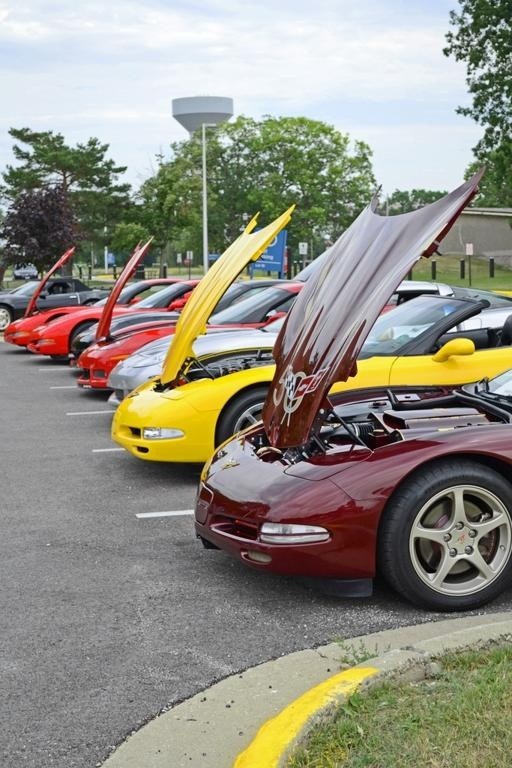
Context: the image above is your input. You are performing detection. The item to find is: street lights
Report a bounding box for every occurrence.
[200,122,218,272]
